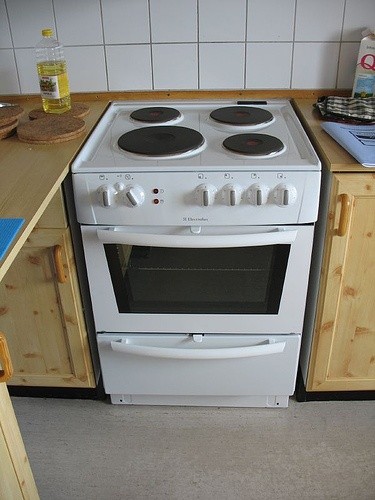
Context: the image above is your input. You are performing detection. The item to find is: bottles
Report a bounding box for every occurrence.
[35,28,72,113]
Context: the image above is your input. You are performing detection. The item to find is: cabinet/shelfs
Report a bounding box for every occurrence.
[0,100,112,500]
[294,97,375,402]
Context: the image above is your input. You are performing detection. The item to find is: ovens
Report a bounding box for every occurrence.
[78,223,313,409]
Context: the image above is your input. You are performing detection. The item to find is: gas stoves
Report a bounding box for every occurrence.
[71,101,320,225]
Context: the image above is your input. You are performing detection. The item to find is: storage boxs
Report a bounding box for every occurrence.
[352,34,375,98]
